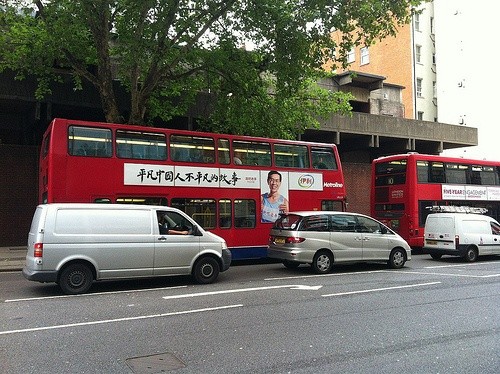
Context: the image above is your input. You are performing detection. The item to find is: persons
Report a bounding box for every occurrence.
[260,170,289,223]
[159,218,191,235]
[313,155,329,168]
[233,152,243,166]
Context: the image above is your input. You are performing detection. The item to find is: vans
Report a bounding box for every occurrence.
[22,203,231,294]
[422,213,500,262]
[268,211,411,274]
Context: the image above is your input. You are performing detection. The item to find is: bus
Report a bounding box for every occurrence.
[370,152,500,252]
[39,117,346,261]
